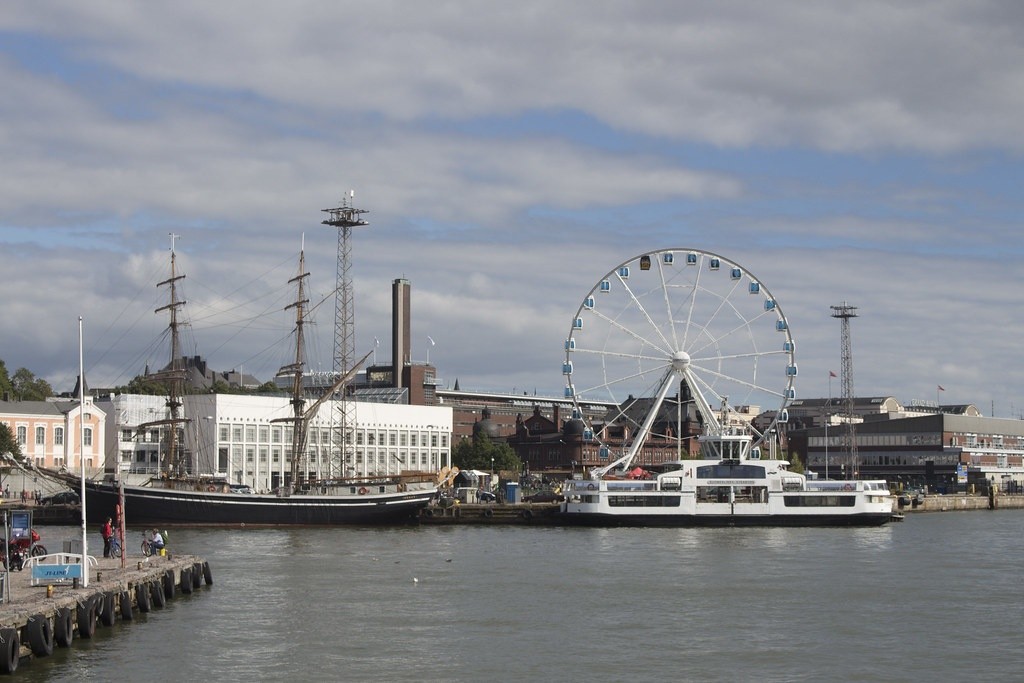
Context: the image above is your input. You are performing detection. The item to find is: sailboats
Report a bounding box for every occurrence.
[549,394,896,527]
[1,187,455,525]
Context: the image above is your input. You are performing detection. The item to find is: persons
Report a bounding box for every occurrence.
[5,483,11,499]
[102,517,114,558]
[476,490,482,504]
[148,528,164,556]
[0,482,3,497]
[34,488,42,505]
[543,476,564,488]
[21,489,27,503]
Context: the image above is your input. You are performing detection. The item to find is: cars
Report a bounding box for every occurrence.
[521,490,564,503]
[480,491,496,501]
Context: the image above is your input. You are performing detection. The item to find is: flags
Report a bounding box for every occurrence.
[938,386,945,390]
[830,370,837,377]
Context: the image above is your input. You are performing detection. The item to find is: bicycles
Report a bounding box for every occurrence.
[108,532,123,559]
[141,532,152,557]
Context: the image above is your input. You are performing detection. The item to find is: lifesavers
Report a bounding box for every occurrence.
[522,508,534,520]
[358,488,367,495]
[0,562,215,675]
[844,483,852,489]
[207,485,216,492]
[484,507,495,518]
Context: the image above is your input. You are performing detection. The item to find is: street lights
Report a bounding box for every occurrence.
[371,335,380,366]
[559,439,567,469]
[825,422,831,482]
[490,456,495,493]
[460,434,468,457]
[426,333,436,367]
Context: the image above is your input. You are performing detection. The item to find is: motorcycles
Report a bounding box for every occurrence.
[2,528,24,576]
[6,528,48,563]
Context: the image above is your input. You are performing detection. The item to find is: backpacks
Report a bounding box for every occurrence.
[157,530,168,547]
[100,524,105,534]
